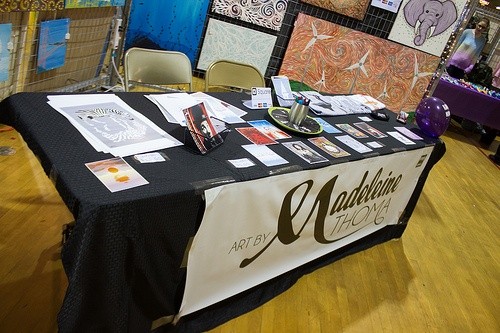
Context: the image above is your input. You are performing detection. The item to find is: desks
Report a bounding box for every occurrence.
[0,92,446,333]
[428,72,500,148]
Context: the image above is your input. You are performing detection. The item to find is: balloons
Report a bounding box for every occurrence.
[416,97,450,138]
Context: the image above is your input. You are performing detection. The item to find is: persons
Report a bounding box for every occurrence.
[445,17,489,78]
[488,144,500,166]
[292,143,324,164]
[190,106,221,150]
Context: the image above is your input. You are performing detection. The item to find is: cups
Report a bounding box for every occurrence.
[287,97,311,125]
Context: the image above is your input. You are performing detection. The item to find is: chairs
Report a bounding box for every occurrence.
[124,47,192,93]
[205,61,265,93]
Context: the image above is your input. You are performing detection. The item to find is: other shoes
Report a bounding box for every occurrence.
[489,154,500,166]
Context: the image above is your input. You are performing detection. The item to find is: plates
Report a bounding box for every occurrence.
[268,107,323,134]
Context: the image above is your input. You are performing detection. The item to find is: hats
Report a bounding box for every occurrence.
[192,106,207,124]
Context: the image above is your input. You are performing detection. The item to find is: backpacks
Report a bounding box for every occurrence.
[467,61,494,91]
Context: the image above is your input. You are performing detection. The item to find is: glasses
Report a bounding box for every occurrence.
[477,24,486,29]
